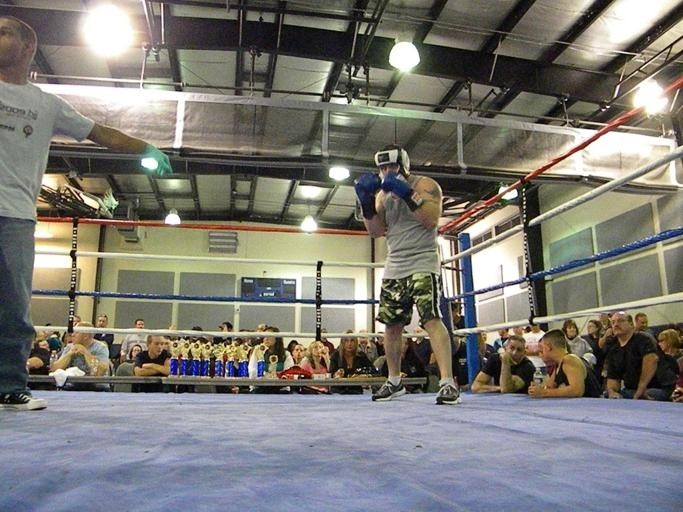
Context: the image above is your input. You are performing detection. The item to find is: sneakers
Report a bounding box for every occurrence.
[434,382,463,405]
[1,391,49,411]
[371,375,407,402]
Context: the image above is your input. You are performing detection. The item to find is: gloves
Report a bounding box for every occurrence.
[354,172,382,219]
[381,173,424,213]
[142,145,173,176]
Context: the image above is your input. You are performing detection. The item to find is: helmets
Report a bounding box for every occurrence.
[373,143,412,178]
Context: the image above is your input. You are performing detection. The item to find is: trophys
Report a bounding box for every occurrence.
[169,339,278,377]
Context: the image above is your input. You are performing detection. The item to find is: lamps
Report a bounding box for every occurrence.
[327,165,350,184]
[497,185,520,201]
[389,32,421,73]
[300,199,319,234]
[164,191,182,226]
[631,78,668,116]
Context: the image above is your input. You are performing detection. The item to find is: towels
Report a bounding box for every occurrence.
[48,366,86,388]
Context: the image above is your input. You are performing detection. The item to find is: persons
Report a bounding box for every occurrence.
[248,327,295,393]
[120,318,150,362]
[300,341,329,393]
[412,327,434,366]
[561,320,592,357]
[376,331,385,357]
[291,345,307,378]
[521,316,546,374]
[355,145,460,406]
[581,320,603,354]
[537,338,559,376]
[357,330,378,362]
[330,329,372,378]
[672,386,683,401]
[581,353,597,368]
[27,324,67,375]
[73,315,80,327]
[605,311,676,402]
[479,324,521,348]
[599,313,612,377]
[1,16,173,410]
[94,315,114,347]
[288,341,297,352]
[382,331,425,376]
[451,301,466,329]
[471,335,536,394]
[130,346,142,360]
[133,335,171,376]
[658,329,680,358]
[320,329,334,352]
[528,330,603,398]
[53,322,112,391]
[635,313,648,331]
[186,322,253,343]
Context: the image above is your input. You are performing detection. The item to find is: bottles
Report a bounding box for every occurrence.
[49,350,57,370]
[532,367,543,400]
[25,361,30,377]
[90,355,99,376]
